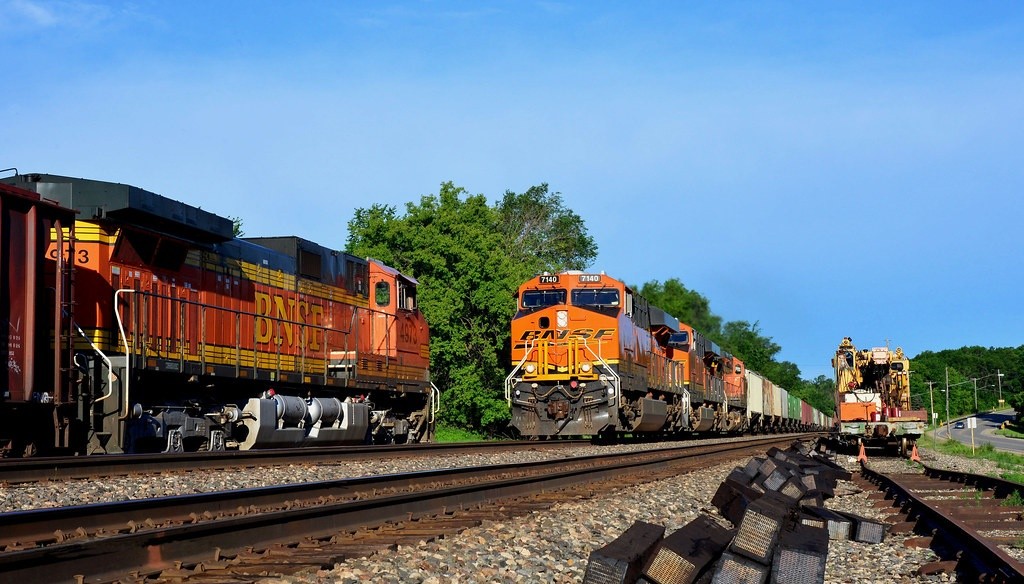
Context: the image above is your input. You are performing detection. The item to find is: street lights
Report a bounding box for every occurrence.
[970,377,984,409]
[924,380,942,425]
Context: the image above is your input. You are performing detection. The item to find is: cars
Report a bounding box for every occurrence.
[955,422,964,429]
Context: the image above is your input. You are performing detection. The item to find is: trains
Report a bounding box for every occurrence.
[0,168,439,459]
[831,337,927,458]
[505,271,832,446]
